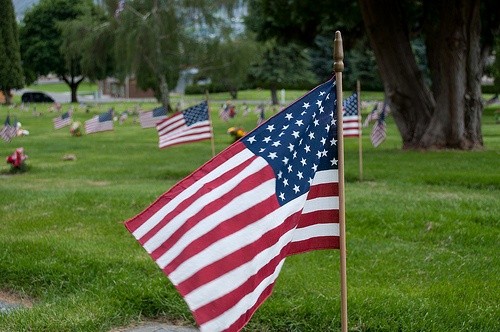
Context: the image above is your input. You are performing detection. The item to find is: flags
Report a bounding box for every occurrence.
[84,111,114,134]
[139,107,167,129]
[342,92,359,138]
[51,111,72,130]
[121,75,340,332]
[364,96,391,147]
[219,99,287,127]
[6,98,85,118]
[1,113,20,144]
[155,100,212,150]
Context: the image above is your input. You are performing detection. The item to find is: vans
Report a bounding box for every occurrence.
[22,92,55,102]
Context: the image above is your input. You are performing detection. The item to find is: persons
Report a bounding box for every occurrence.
[276,78,286,107]
